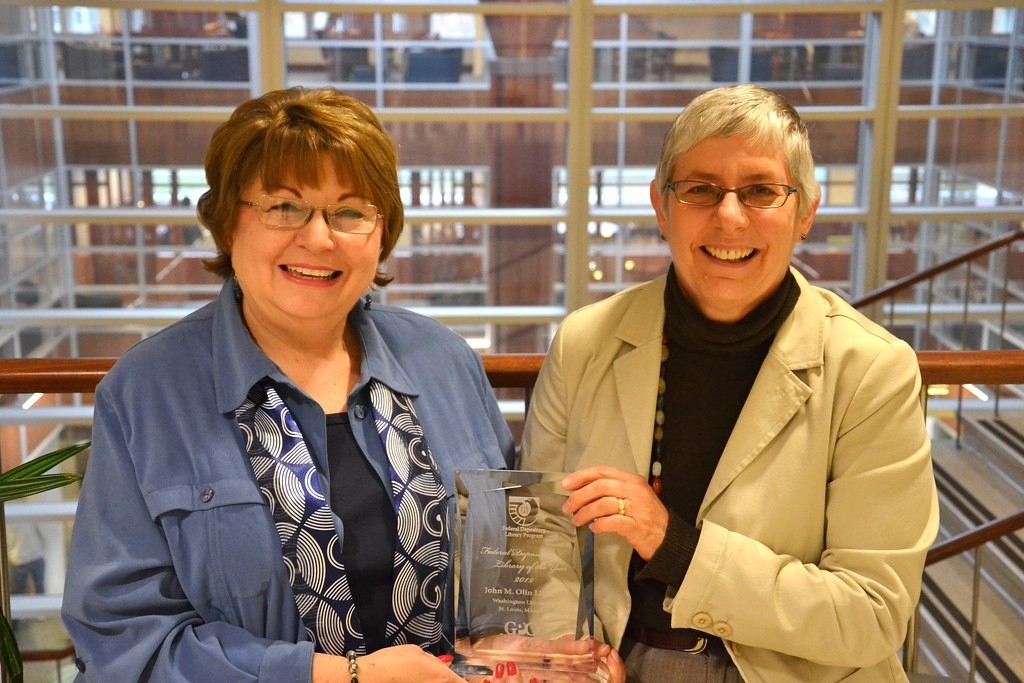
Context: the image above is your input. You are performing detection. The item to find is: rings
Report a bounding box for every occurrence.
[618,498,625,515]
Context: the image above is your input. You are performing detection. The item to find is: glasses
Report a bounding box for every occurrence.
[666,179,803,209]
[238,194,385,234]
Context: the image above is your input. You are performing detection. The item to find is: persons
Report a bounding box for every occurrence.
[5,519,47,596]
[509,83,942,683]
[59,84,595,683]
[223,10,249,82]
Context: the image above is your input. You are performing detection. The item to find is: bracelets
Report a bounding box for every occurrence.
[346,650,359,683]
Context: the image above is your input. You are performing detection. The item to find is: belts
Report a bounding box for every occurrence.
[624,626,723,656]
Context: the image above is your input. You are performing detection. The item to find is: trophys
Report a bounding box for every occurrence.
[436,466,613,683]
